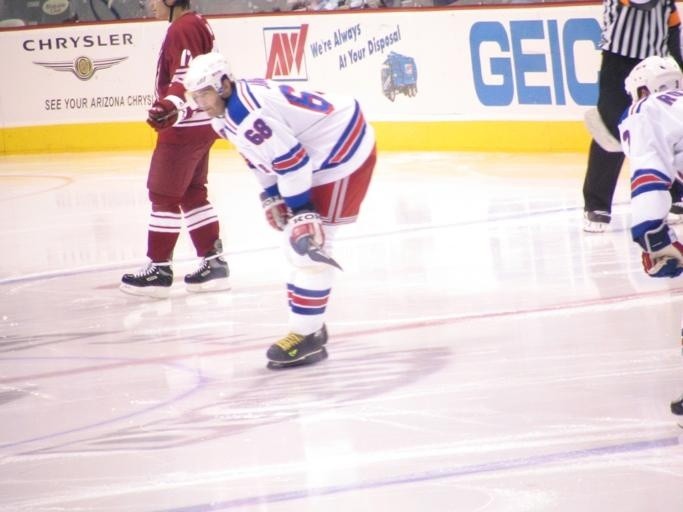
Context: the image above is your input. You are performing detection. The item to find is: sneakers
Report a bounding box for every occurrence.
[267,322,326,360]
[184,254,229,284]
[122,262,173,286]
[583,209,610,223]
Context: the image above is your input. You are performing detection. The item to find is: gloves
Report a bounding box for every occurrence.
[147,106,167,128]
[288,213,329,256]
[642,223,683,277]
[260,190,288,232]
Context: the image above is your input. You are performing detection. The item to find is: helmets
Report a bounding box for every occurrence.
[624,56,683,103]
[183,53,233,93]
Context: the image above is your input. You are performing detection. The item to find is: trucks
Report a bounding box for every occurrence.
[380,52,419,101]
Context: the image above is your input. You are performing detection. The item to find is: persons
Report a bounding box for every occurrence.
[181,50,379,371]
[616,54,683,419]
[119,1,230,288]
[582,0,683,232]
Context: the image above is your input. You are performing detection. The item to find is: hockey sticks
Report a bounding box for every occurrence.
[156,97,196,122]
[307,240,343,270]
[583,107,623,153]
